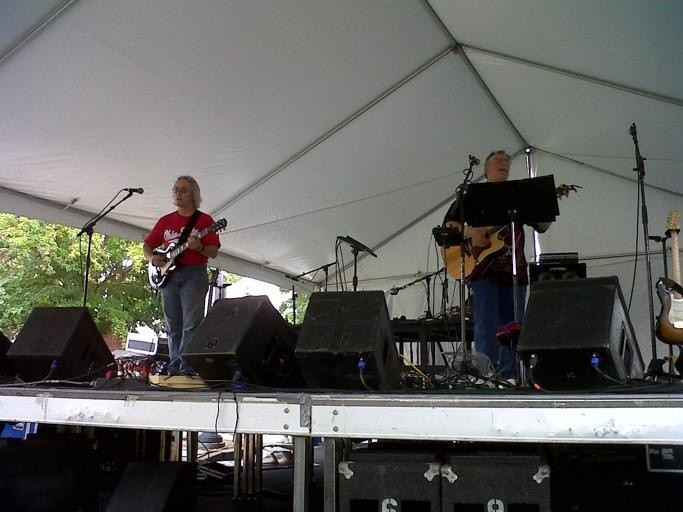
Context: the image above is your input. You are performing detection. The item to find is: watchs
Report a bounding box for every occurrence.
[195,244,204,253]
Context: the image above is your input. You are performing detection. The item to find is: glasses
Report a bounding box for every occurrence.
[172,187,191,194]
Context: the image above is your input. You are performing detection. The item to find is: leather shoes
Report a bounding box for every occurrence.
[181,369,196,376]
[167,371,180,376]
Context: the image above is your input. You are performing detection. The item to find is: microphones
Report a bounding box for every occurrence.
[627,122,637,135]
[468,154,480,165]
[124,188,143,194]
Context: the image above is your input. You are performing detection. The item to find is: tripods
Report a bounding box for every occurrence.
[435,161,514,390]
[390,267,451,369]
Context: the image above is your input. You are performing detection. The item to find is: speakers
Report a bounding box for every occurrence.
[294,290,402,392]
[104,460,199,512]
[5,306,118,388]
[515,274,645,392]
[180,295,299,389]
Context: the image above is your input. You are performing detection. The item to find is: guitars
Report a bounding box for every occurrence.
[147,218,227,290]
[656,210,683,344]
[441,184,583,282]
[207,268,220,314]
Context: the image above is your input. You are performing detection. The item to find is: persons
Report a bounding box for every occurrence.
[444,147,552,392]
[114,356,167,379]
[141,175,220,377]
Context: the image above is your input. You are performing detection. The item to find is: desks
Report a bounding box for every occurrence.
[441,445,551,512]
[338,444,442,512]
